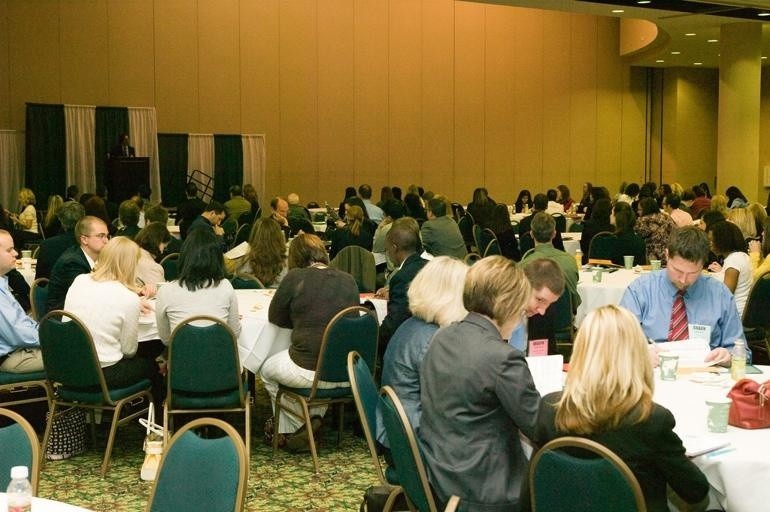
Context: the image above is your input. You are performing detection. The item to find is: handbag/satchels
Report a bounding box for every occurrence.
[138,401,172,481]
[43,404,89,460]
[727,376,770,430]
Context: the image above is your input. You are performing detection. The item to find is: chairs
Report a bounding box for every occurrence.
[20,414,43,497]
[31,309,159,481]
[159,252,180,281]
[587,231,617,261]
[552,286,574,343]
[0,372,53,412]
[146,418,250,504]
[509,219,521,234]
[29,278,52,322]
[165,315,252,481]
[551,212,586,232]
[221,217,251,248]
[111,217,120,229]
[31,244,42,260]
[230,272,266,290]
[251,206,263,225]
[271,306,379,476]
[458,208,502,267]
[521,248,536,261]
[307,200,328,223]
[528,437,648,512]
[347,350,464,512]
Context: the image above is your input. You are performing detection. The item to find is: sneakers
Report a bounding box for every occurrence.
[263,416,287,449]
[287,417,323,450]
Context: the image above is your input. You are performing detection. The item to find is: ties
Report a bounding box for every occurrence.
[668,291,690,342]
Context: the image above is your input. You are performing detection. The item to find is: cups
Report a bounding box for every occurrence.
[622,254,634,268]
[707,395,732,433]
[20,249,33,272]
[659,353,677,381]
[593,267,604,283]
[650,259,662,270]
[157,283,164,289]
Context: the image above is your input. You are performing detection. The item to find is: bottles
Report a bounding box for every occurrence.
[511,204,516,215]
[6,464,33,512]
[574,249,582,269]
[571,204,576,216]
[525,204,529,214]
[730,339,746,380]
